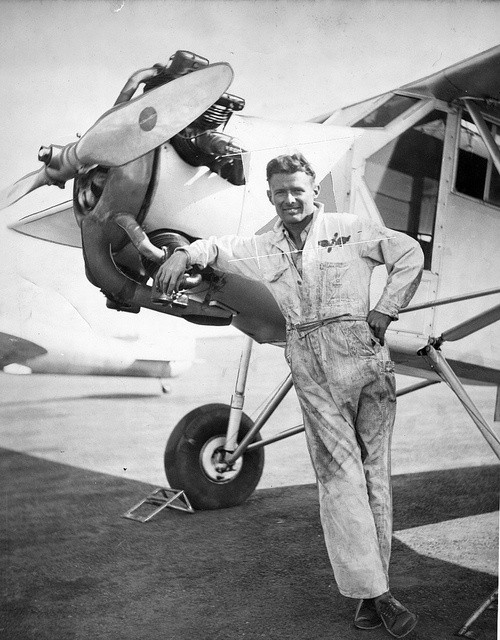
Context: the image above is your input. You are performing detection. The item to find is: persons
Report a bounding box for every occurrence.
[155,152,428,639]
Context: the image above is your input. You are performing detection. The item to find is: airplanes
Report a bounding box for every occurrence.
[0,330,191,393]
[0,45,500,521]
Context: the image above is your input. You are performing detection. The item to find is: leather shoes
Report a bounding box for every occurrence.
[376,596,417,638]
[354,599,383,629]
